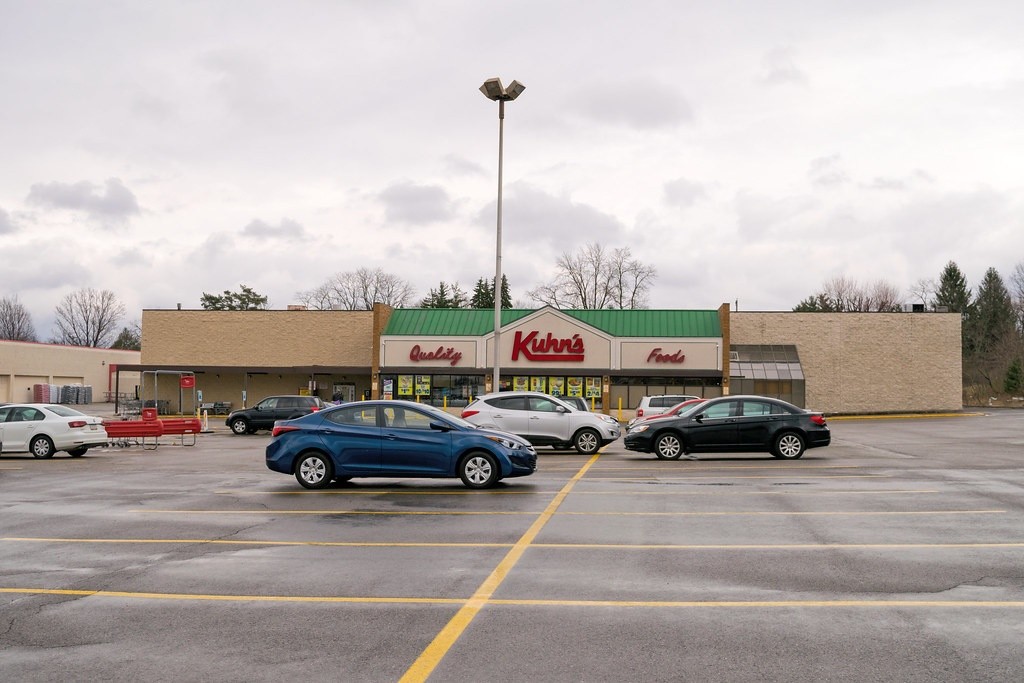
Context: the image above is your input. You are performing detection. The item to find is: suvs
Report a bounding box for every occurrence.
[461,391,622,455]
[636,394,701,419]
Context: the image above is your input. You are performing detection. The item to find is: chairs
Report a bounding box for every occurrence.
[336,415,346,423]
[346,412,357,423]
[34,410,44,420]
[729,402,737,417]
[393,411,409,427]
[13,412,23,421]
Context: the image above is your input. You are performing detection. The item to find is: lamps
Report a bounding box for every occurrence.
[217,374,220,378]
[342,375,346,381]
[308,375,311,380]
[278,375,282,380]
[248,375,252,380]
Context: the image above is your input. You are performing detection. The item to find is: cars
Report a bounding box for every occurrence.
[0,404,107,459]
[323,402,338,408]
[628,399,771,430]
[626,395,831,459]
[266,397,538,489]
[225,396,326,435]
[441,392,450,399]
[534,397,589,449]
[422,394,434,400]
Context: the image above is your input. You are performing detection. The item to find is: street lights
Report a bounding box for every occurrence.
[480,78,527,393]
